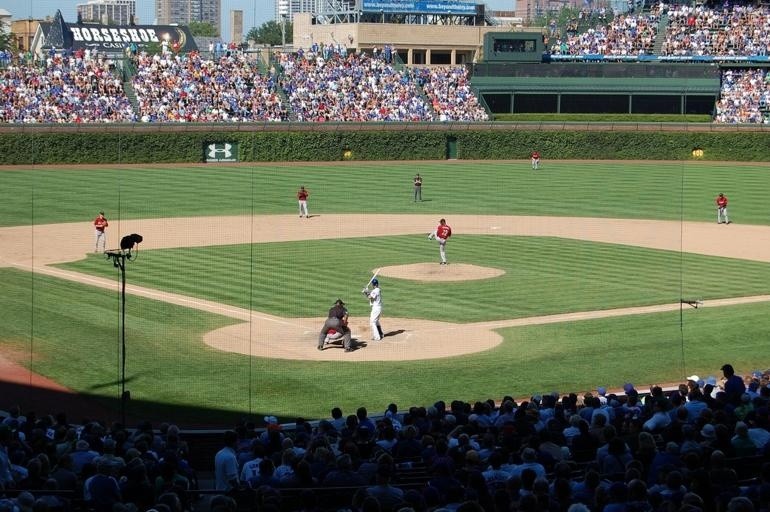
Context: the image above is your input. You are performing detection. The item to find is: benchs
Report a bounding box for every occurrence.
[634,9,770,123]
[0,407,770,512]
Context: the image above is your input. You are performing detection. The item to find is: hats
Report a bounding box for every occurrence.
[301,186,304,189]
[438,219,445,222]
[100,211,104,215]
[334,299,345,305]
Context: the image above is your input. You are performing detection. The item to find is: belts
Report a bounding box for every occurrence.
[439,237,446,239]
[328,316,338,319]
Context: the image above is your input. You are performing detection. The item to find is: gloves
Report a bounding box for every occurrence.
[363,287,370,295]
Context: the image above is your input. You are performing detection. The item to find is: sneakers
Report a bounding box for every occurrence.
[427,233,432,240]
[440,261,447,266]
[317,345,323,351]
[344,348,352,352]
[370,335,383,340]
[299,215,309,218]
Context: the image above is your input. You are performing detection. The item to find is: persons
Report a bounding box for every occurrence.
[324,321,348,345]
[296,185,310,218]
[715,193,729,224]
[364,278,384,341]
[0,33,491,126]
[531,150,540,170]
[94,211,108,252]
[316,299,352,352]
[413,173,422,202]
[0,363,770,512]
[713,66,770,128]
[536,0,770,63]
[428,218,451,264]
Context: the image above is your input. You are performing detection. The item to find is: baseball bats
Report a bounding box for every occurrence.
[361,267,381,294]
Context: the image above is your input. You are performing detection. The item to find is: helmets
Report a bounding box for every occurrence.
[372,279,378,285]
